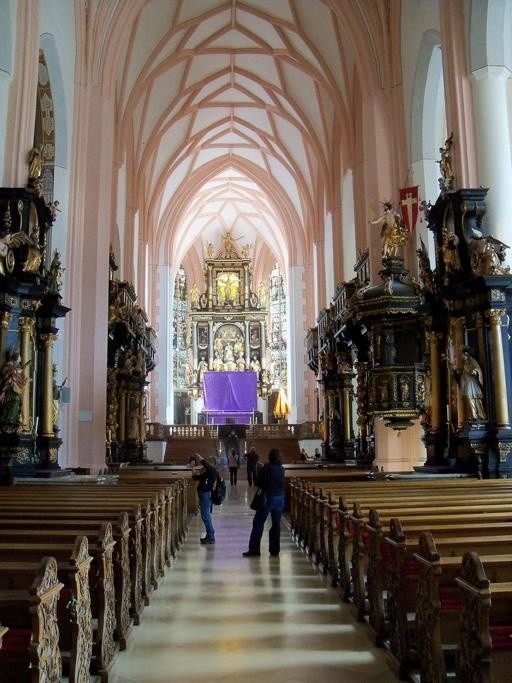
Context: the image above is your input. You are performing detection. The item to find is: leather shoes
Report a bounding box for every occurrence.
[270,550,279,556]
[242,551,260,555]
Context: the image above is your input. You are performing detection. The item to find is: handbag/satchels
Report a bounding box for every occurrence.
[250,495,265,511]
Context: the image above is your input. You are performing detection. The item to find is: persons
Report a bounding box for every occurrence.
[241,447,285,557]
[50,253,65,292]
[28,144,44,178]
[2,367,21,434]
[191,452,216,545]
[227,447,240,487]
[300,448,321,462]
[244,446,259,487]
[368,201,401,259]
[450,349,486,420]
[196,334,261,382]
[51,200,63,219]
[439,148,453,179]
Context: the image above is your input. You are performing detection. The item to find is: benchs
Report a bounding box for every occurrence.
[1,466,200,681]
[285,468,512,683]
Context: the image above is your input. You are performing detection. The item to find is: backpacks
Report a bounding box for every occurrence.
[211,476,226,506]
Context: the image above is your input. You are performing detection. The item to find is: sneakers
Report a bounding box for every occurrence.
[200,536,216,544]
